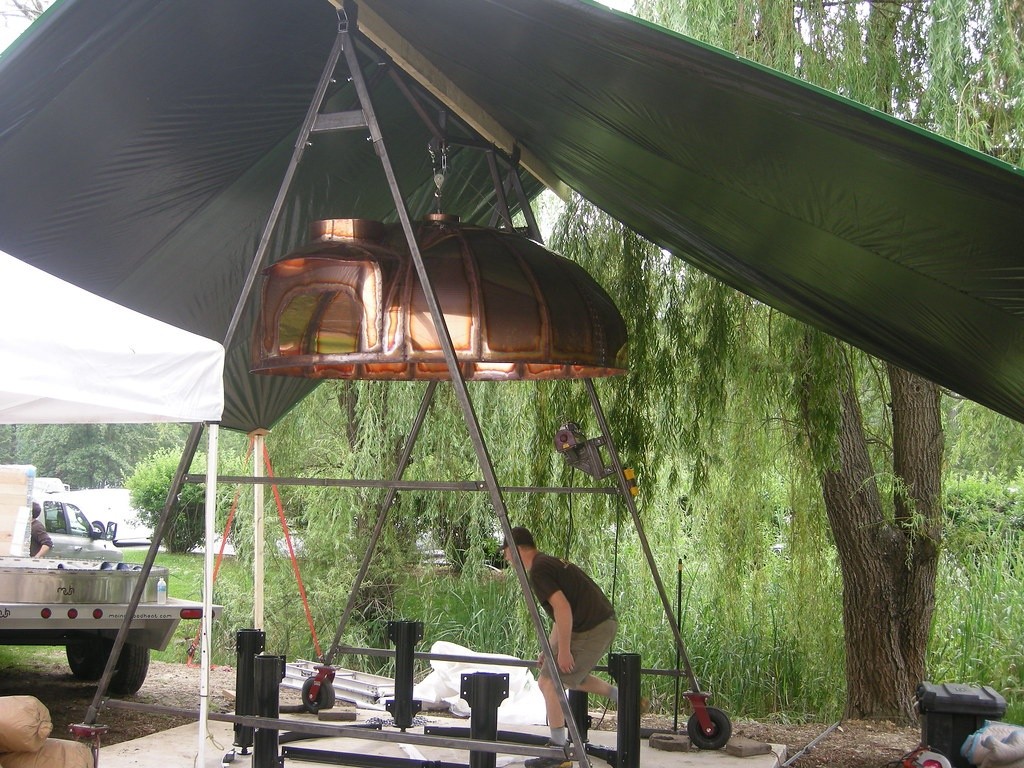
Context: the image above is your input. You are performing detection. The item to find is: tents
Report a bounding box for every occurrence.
[0,252,266,767]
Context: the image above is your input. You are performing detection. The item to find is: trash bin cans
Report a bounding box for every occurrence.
[916,680,1007,768]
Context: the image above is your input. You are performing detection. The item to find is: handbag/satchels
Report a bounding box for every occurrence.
[881,745,952,768]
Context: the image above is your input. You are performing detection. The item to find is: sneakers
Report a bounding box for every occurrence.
[524,739,573,768]
[640,695,649,716]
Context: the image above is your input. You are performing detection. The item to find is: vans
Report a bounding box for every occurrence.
[29,492,125,565]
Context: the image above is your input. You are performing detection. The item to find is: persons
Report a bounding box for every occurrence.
[31,502,53,558]
[504,528,651,768]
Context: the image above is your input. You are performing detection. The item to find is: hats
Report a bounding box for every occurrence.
[496,527,534,554]
